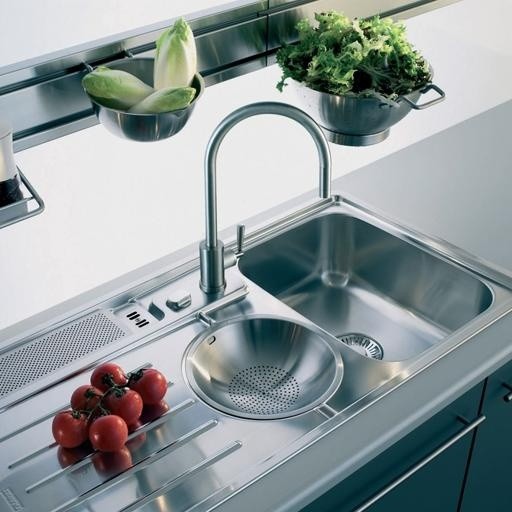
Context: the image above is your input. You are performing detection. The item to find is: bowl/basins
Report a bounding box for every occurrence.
[287,53,446,147]
[83,56,204,142]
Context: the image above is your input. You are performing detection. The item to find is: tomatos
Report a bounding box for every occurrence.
[108,387,143,426]
[141,401,169,425]
[90,364,126,394]
[91,450,131,477]
[126,422,146,449]
[70,385,103,412]
[89,405,128,451]
[57,447,86,469]
[128,368,165,403]
[52,409,90,449]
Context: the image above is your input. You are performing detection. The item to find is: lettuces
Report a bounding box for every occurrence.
[276,9,431,108]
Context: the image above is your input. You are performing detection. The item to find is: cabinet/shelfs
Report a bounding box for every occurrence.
[298,356,512,512]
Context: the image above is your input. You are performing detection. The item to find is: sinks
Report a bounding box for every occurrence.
[237,194,512,365]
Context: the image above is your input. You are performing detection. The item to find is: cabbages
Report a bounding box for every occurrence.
[81,66,154,109]
[128,86,196,115]
[153,17,196,92]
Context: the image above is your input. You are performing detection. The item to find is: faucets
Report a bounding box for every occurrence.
[200,102,331,293]
